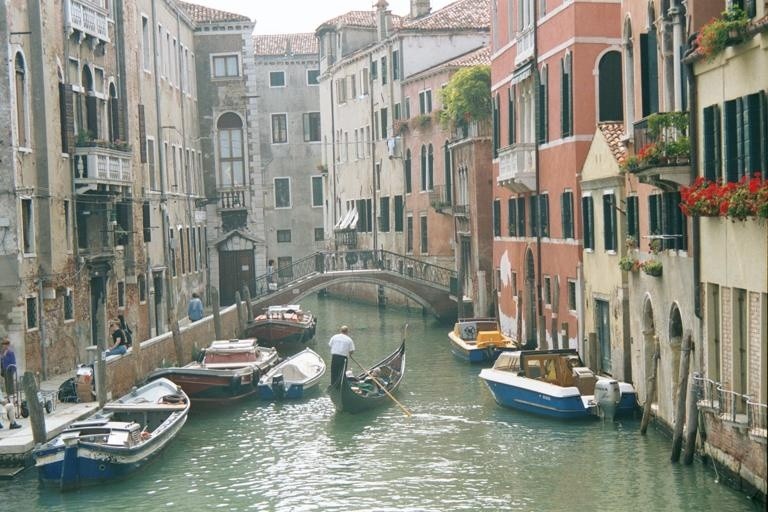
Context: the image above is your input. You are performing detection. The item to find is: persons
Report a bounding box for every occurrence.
[328,325,354,384]
[0,390,21,429]
[266,259,274,284]
[0,339,17,399]
[188,293,203,323]
[105,317,126,357]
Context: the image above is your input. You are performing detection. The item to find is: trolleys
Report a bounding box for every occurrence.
[16,370,53,419]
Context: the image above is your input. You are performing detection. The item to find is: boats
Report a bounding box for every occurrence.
[146,337,279,409]
[447,317,516,365]
[477,346,638,423]
[34,377,190,492]
[256,346,327,401]
[327,323,409,414]
[242,303,318,346]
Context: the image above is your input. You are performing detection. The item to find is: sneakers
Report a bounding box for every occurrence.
[9,421,21,429]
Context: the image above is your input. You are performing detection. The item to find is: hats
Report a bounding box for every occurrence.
[111,317,121,324]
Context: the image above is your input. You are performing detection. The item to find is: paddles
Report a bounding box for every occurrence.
[348,353,413,417]
